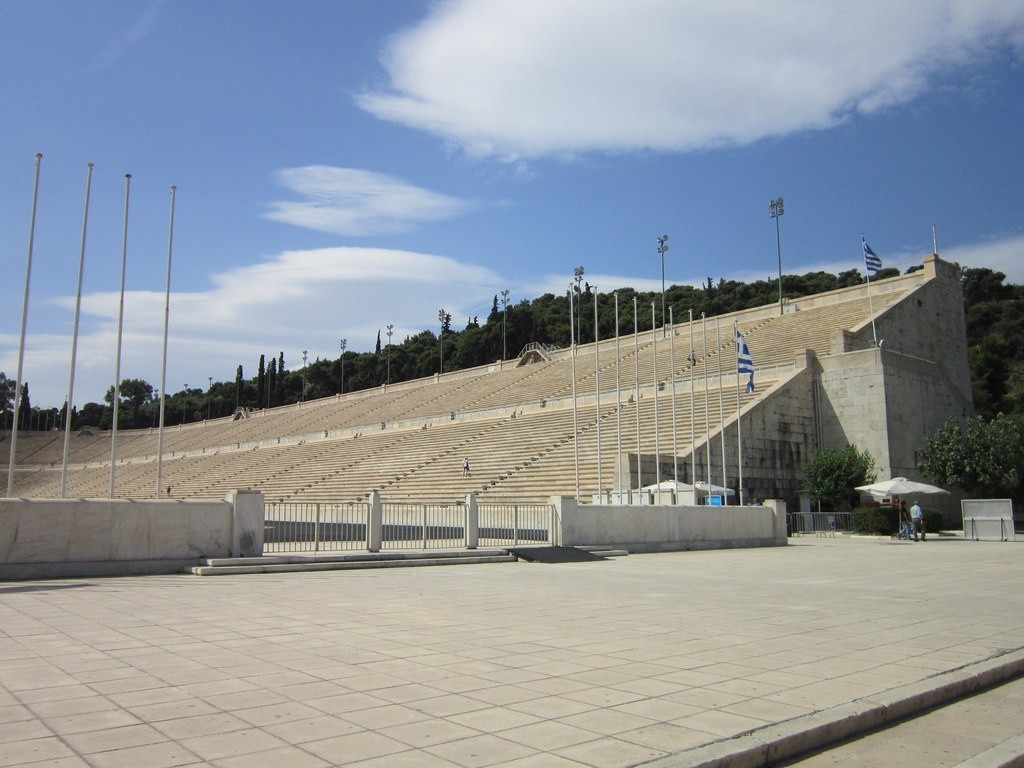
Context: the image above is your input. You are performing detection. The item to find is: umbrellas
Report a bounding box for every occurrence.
[854,476,950,532]
[636,480,735,505]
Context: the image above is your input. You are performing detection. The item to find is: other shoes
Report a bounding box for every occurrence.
[920,539,927,541]
[897,533,901,540]
[914,540,919,542]
[902,535,907,540]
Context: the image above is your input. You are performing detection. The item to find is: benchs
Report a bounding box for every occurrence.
[0,288,911,511]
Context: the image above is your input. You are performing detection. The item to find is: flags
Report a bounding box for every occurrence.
[863,239,882,272]
[736,332,754,394]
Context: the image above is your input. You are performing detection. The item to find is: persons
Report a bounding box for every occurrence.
[909,501,926,542]
[462,457,469,478]
[747,497,761,506]
[897,500,913,540]
[689,350,696,366]
[167,486,171,497]
